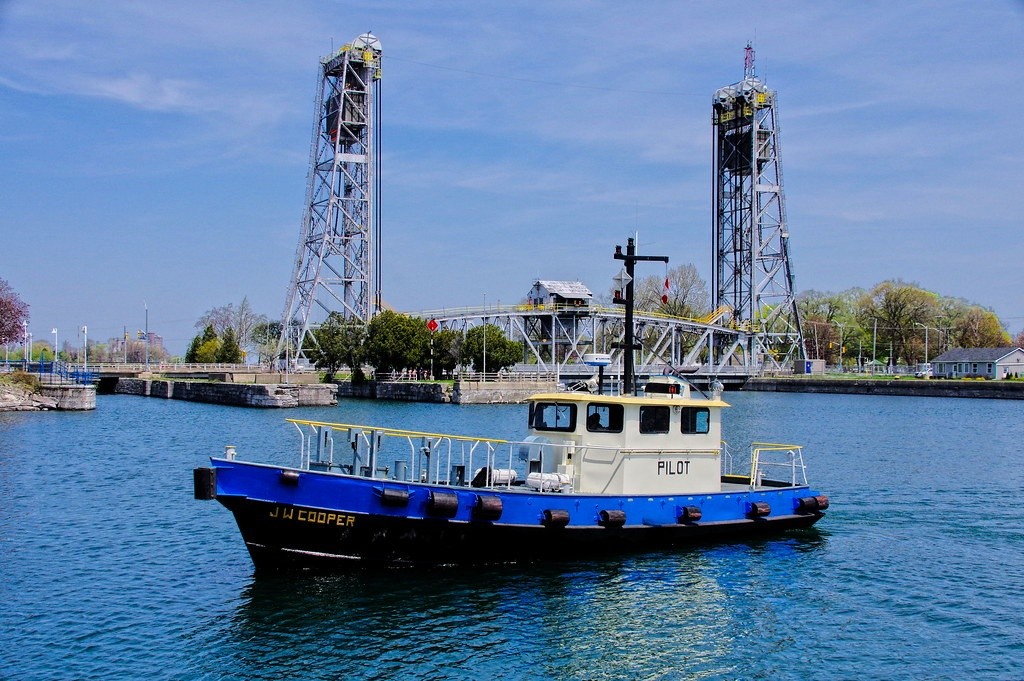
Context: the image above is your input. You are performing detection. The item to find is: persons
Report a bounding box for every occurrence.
[589,412,602,430]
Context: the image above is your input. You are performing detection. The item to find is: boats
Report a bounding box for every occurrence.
[191,228,829,566]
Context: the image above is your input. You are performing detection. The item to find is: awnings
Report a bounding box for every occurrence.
[558,293,593,298]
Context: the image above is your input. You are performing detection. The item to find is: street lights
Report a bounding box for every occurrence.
[914,322,928,379]
[22,320,32,374]
[831,319,843,372]
[869,316,878,379]
[81,326,87,372]
[51,327,59,372]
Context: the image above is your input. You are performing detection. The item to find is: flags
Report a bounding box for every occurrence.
[661,279,671,305]
